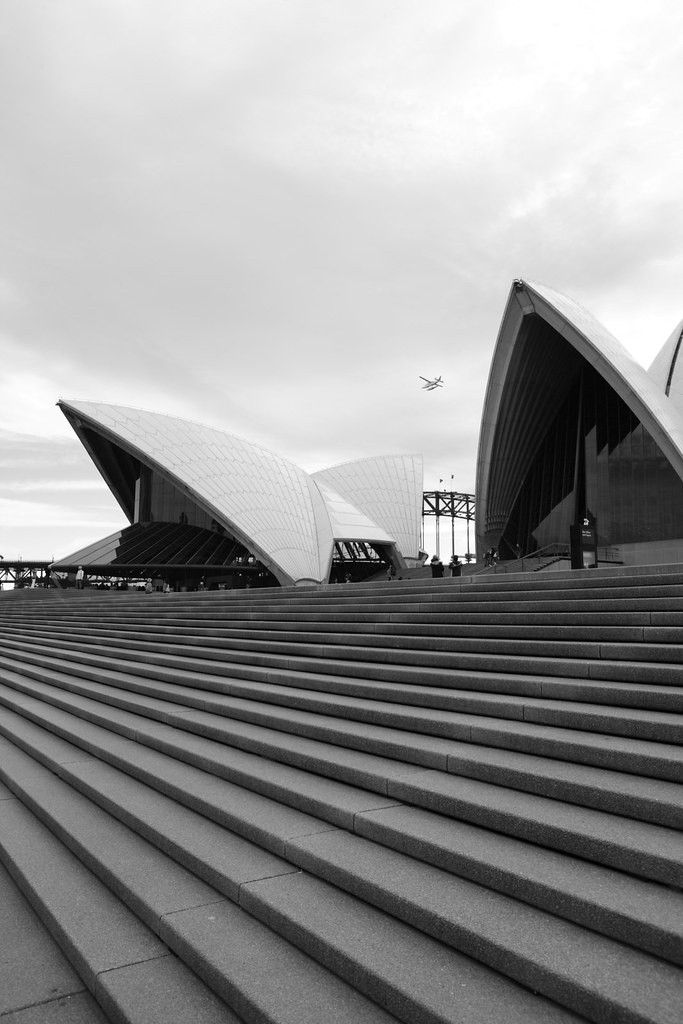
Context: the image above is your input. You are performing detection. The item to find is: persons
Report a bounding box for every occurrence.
[482,546,498,567]
[448,555,462,577]
[516,543,521,559]
[200,576,204,591]
[162,579,170,593]
[430,555,444,578]
[345,573,352,583]
[75,566,84,589]
[386,565,397,581]
[145,582,153,594]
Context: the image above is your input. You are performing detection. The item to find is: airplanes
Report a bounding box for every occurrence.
[419,376,443,391]
